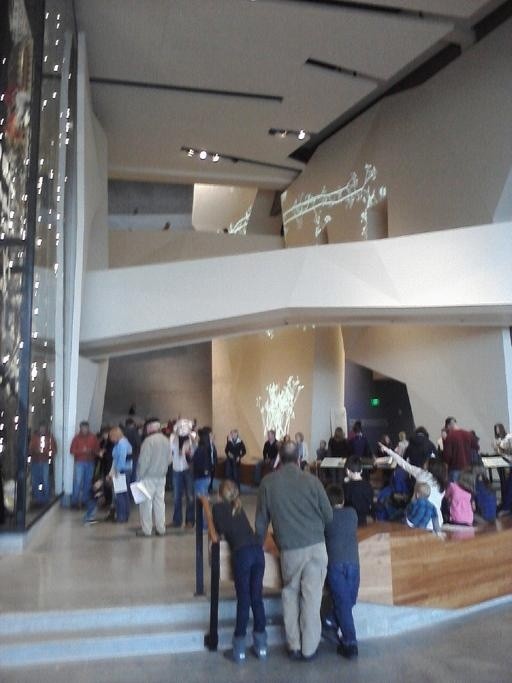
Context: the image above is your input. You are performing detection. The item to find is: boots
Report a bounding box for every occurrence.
[224,637,245,663]
[250,632,268,661]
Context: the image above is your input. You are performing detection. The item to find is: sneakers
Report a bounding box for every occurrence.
[288,649,318,661]
[137,523,193,535]
[337,644,358,657]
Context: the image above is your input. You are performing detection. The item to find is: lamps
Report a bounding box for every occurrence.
[182,146,222,163]
[268,127,306,140]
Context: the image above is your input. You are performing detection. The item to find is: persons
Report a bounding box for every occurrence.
[318,417,512,533]
[321,486,360,658]
[28,421,57,508]
[262,431,307,475]
[256,440,333,662]
[212,478,268,663]
[71,418,247,537]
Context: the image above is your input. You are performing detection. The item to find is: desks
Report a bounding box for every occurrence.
[193,492,283,652]
[320,455,512,520]
